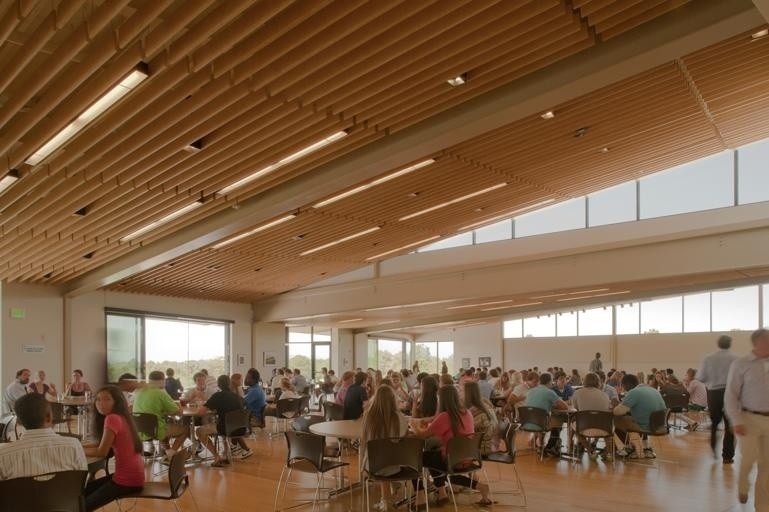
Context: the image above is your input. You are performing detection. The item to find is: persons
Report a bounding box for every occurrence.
[1,353,267,511]
[722,329,769,512]
[695,334,741,464]
[264,360,501,511]
[489,352,707,459]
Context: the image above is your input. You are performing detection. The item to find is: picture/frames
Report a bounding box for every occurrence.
[461,357,470,368]
[479,357,491,368]
[263,351,278,367]
[237,353,247,367]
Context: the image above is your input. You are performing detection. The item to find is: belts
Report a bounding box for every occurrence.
[740,406,769,420]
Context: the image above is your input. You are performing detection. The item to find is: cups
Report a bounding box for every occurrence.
[402,415,425,429]
[173,397,186,410]
[57,391,66,401]
[85,391,95,400]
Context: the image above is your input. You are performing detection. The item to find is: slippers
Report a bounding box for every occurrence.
[474,498,499,506]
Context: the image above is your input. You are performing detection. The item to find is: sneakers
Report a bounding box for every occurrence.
[407,496,450,512]
[722,457,735,464]
[236,447,255,460]
[617,446,657,460]
[210,458,232,468]
[229,447,242,456]
[739,493,748,504]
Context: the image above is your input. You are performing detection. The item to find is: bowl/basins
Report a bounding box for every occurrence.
[187,401,204,410]
